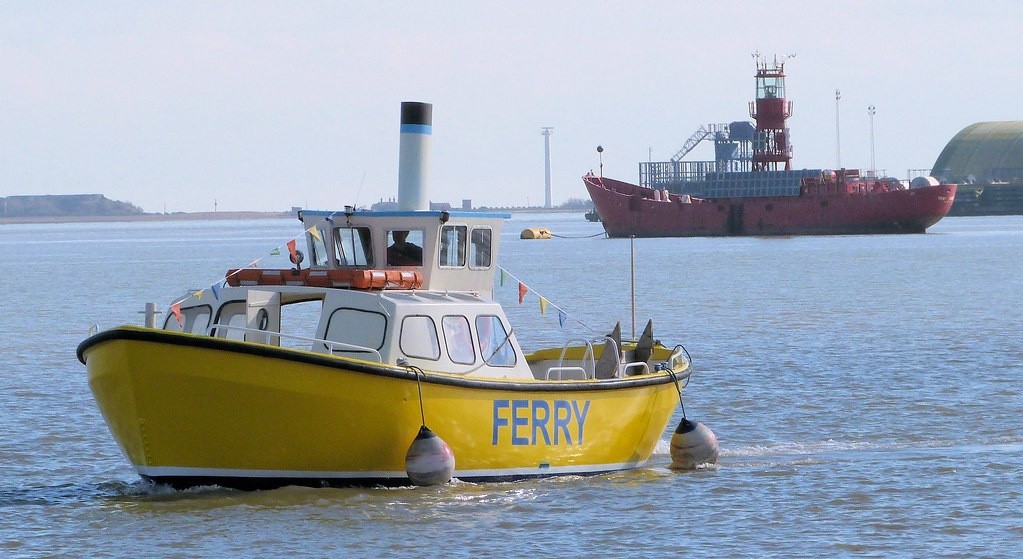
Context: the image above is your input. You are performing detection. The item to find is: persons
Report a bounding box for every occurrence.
[387,230,422,266]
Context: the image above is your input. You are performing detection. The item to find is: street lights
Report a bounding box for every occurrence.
[835,89,840,168]
[866,105,875,169]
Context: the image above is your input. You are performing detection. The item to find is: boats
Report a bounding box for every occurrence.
[582,52,960,236]
[75,100,694,491]
